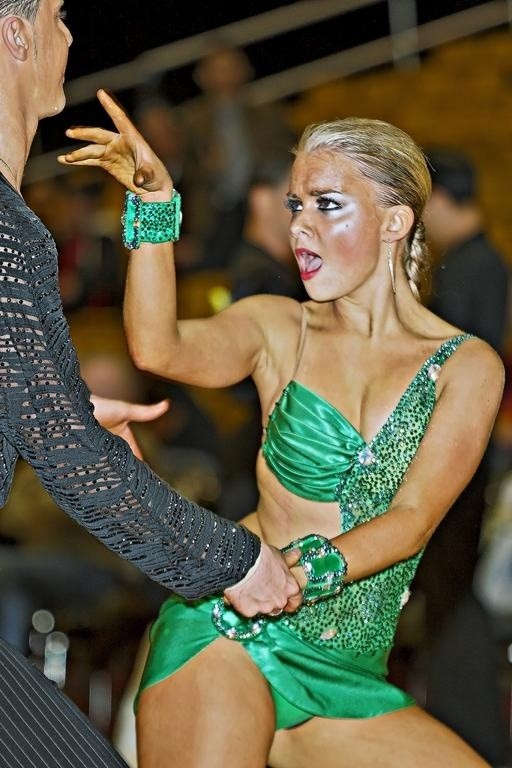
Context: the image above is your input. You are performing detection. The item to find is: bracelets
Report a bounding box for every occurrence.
[122,190,181,250]
[282,531,349,605]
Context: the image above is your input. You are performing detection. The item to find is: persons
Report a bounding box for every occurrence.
[227,134,311,409]
[0,0,305,768]
[411,140,512,640]
[139,48,296,282]
[53,89,507,768]
